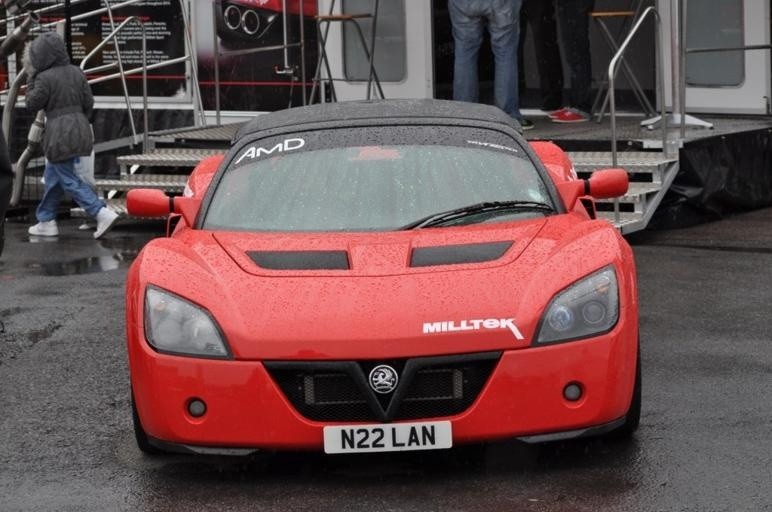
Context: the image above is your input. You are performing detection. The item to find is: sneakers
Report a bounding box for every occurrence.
[519,119,535,130]
[93,207,120,239]
[548,109,589,123]
[28,219,59,236]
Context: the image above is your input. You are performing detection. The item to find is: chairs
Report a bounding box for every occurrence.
[587,0,657,124]
[308,0,385,105]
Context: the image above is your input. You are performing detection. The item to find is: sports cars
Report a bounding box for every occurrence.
[125,98,641,460]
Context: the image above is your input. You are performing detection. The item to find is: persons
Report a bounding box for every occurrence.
[22,32,120,240]
[447,0,595,131]
